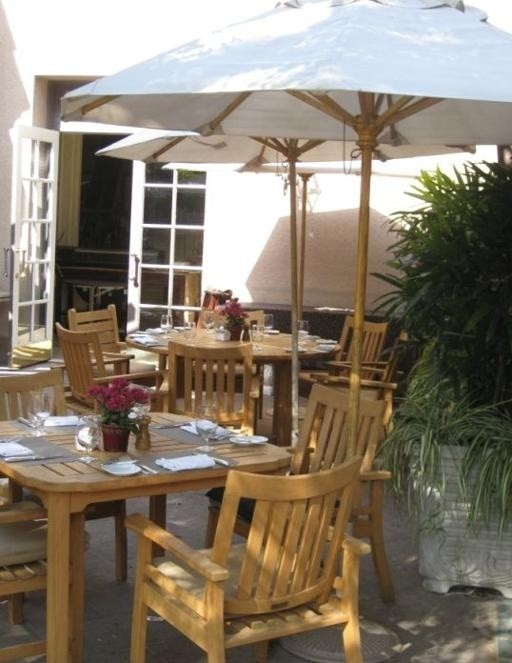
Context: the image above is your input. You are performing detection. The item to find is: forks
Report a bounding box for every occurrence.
[117,454,159,475]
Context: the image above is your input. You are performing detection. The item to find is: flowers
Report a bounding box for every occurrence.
[219,301,251,330]
[80,376,148,437]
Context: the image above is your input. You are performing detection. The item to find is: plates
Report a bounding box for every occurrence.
[284,346,310,351]
[100,460,141,476]
[230,434,269,447]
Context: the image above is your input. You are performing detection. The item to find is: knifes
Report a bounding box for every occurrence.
[192,450,232,467]
[18,414,43,432]
[154,421,188,431]
[234,437,266,445]
[4,453,70,467]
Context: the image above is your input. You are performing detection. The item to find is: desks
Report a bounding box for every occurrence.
[55,267,201,324]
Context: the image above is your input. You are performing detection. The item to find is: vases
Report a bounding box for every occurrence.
[228,325,251,340]
[99,424,130,453]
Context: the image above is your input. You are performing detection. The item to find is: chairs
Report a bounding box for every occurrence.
[298,338,408,388]
[205,383,408,603]
[298,315,390,419]
[55,322,169,414]
[193,307,265,420]
[124,454,371,663]
[168,339,259,436]
[67,304,155,373]
[0,369,127,625]
[326,332,415,375]
[1,477,91,661]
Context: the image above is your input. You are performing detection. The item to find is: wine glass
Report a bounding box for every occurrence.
[26,392,54,436]
[183,322,196,344]
[213,319,224,341]
[201,311,215,334]
[250,325,264,350]
[73,414,103,463]
[129,386,152,436]
[160,315,175,339]
[194,403,220,453]
[262,314,273,337]
[297,320,309,348]
